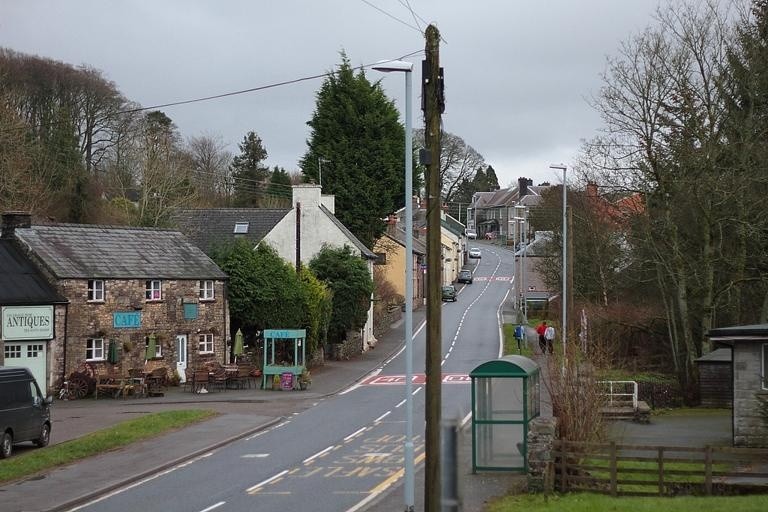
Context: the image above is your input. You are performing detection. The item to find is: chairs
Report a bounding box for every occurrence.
[96,361,261,401]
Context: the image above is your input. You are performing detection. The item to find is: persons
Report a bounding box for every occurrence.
[512,324,526,351]
[543,323,557,355]
[536,321,548,354]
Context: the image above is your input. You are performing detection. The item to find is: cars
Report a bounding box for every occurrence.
[442,286,457,302]
[469,248,481,258]
[458,270,472,284]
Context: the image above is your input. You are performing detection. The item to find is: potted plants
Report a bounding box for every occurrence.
[272,374,280,391]
[296,366,312,391]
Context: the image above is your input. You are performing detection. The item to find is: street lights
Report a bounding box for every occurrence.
[374,59,417,508]
[549,163,567,366]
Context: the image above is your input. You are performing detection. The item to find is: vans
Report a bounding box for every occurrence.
[0,366,53,458]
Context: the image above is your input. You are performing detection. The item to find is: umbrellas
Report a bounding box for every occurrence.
[107,334,118,376]
[144,329,159,361]
[232,328,245,357]
